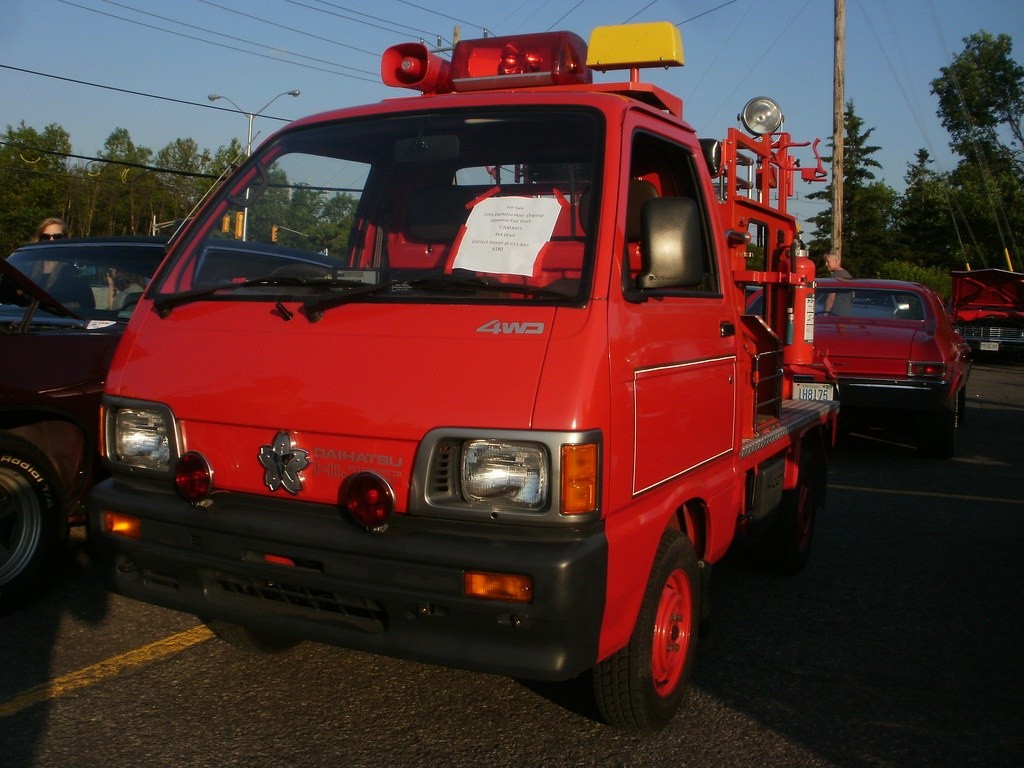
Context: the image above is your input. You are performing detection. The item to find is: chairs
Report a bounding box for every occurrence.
[527,174,660,295]
[355,166,477,271]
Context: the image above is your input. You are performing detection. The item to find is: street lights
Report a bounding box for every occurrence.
[206,90,302,241]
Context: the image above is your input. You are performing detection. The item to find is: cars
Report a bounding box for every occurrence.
[2,234,343,612]
[743,278,974,449]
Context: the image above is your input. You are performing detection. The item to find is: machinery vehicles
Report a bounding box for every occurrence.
[851,0,1024,365]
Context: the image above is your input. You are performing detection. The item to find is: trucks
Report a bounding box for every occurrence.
[84,21,848,740]
